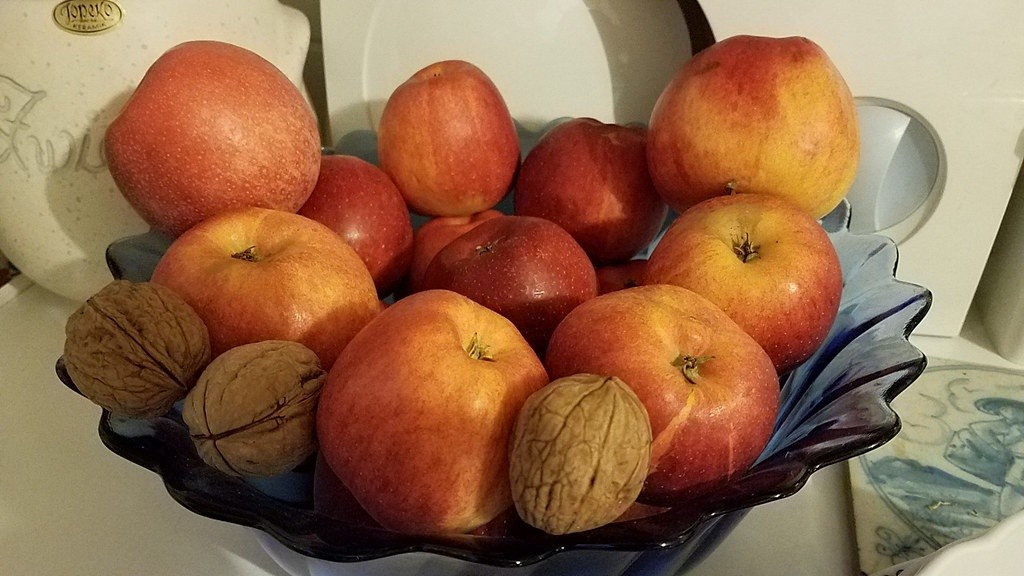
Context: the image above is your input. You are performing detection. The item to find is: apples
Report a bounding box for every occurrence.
[102,31,863,538]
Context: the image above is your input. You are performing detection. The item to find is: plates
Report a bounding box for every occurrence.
[54,116,933,576]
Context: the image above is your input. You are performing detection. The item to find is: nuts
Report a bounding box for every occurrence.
[64,279,212,421]
